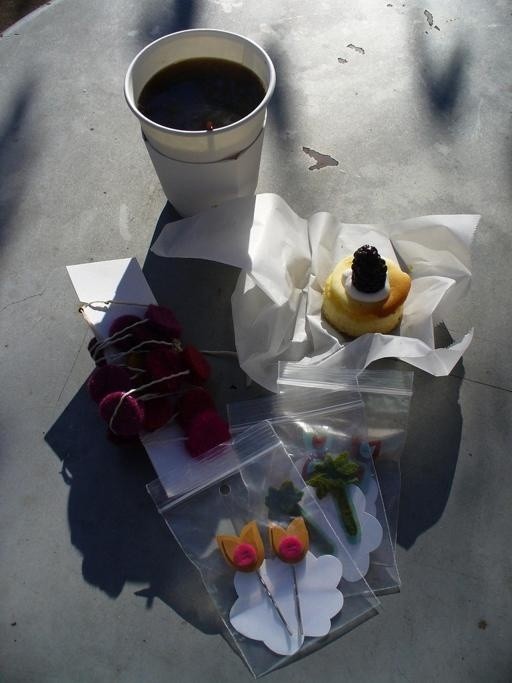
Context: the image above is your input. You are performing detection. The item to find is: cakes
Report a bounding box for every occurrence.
[320,243,413,338]
